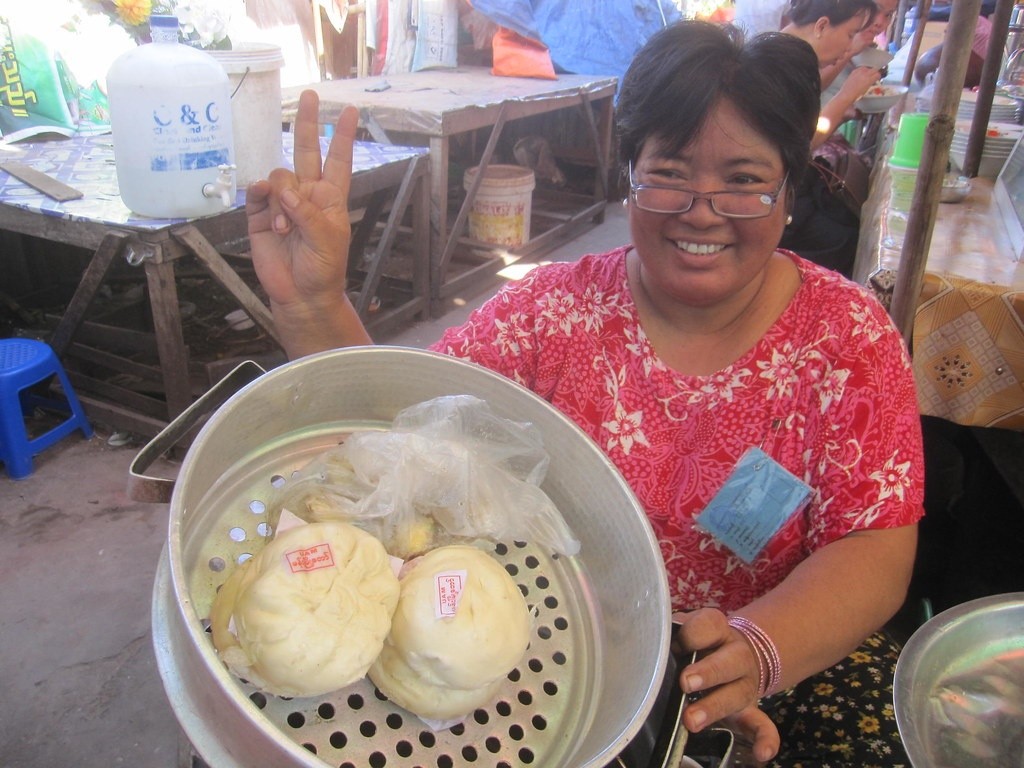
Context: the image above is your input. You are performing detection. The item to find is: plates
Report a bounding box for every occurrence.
[915,89,1020,126]
[949,120,1024,173]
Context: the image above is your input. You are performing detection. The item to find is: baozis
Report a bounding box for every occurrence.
[206,523,534,719]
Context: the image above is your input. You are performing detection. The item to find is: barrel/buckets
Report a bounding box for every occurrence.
[462,163,535,255]
[886,111,930,171]
[209,46,287,190]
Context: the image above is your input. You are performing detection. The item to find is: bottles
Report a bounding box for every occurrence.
[106,16,237,217]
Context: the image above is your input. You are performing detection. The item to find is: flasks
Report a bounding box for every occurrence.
[1005,5,1024,60]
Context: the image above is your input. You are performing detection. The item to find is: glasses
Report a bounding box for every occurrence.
[628,158,790,219]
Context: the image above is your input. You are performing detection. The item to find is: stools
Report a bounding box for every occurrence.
[0,338,94,479]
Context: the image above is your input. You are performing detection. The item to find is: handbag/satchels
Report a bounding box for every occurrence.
[812,146,873,205]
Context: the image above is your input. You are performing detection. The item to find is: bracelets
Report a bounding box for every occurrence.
[725,613,781,706]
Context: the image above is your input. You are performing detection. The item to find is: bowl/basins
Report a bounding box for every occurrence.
[939,175,972,203]
[893,591,1024,768]
[224,308,254,331]
[850,47,895,71]
[852,84,908,114]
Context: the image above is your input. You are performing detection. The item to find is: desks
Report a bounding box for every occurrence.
[0,62,619,463]
[882,22,950,88]
[852,91,1024,525]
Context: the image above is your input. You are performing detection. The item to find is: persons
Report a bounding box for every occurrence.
[910,11,1002,91]
[777,1,899,272]
[245,17,924,768]
